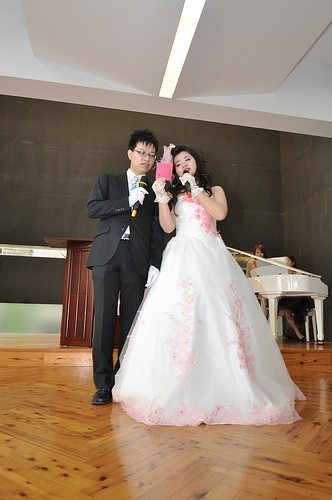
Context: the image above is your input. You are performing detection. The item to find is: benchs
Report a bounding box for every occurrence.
[266,307,316,343]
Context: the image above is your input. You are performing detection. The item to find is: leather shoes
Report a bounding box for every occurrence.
[92,387,112,404]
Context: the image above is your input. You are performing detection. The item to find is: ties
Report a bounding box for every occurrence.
[131,176,139,190]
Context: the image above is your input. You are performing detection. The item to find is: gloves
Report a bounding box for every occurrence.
[145,265,160,288]
[129,187,149,207]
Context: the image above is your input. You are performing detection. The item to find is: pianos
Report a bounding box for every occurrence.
[227,246,328,345]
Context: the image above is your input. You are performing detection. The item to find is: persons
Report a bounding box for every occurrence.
[112,145,306,426]
[85,130,163,404]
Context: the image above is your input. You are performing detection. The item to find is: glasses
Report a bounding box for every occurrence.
[131,149,157,160]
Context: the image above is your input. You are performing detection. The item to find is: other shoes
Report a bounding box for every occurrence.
[295,333,306,342]
